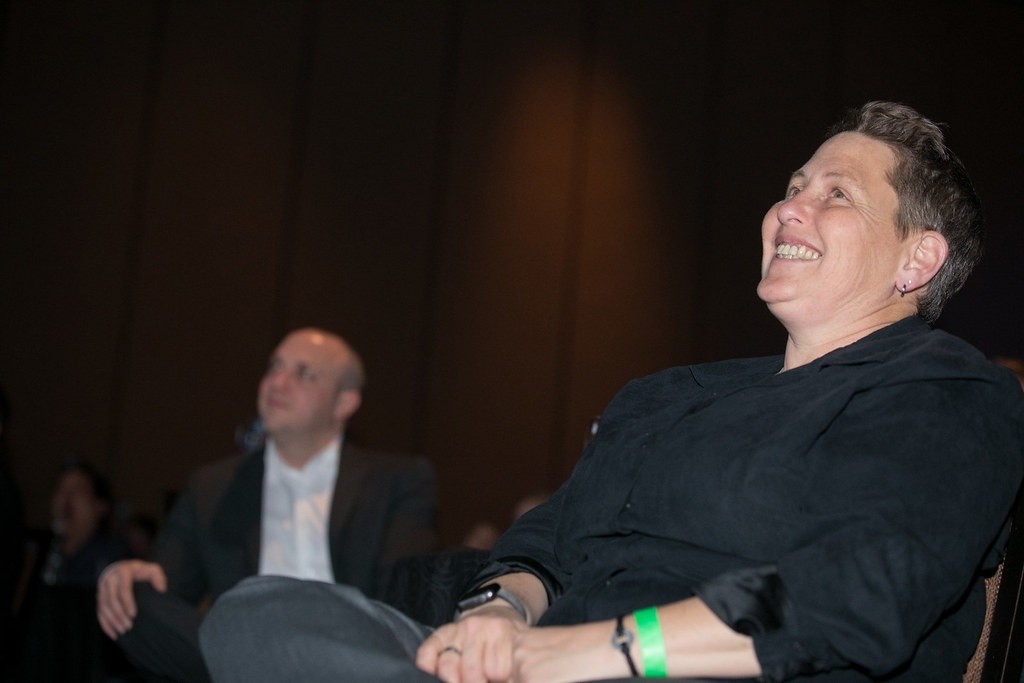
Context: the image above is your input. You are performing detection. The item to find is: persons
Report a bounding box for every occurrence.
[464,496,547,549]
[0,463,157,683]
[196,100,1024,683]
[97,328,442,683]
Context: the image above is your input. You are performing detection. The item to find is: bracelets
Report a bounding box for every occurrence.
[612,606,666,679]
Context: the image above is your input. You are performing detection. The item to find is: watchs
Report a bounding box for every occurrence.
[453,583,527,622]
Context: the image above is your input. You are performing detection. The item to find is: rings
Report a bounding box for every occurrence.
[438,646,461,656]
